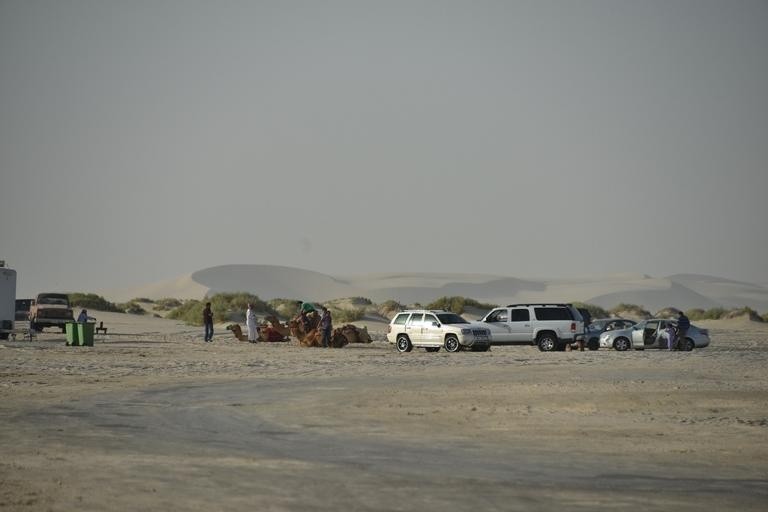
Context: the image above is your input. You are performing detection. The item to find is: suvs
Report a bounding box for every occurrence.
[467,303,591,352]
[386,309,493,353]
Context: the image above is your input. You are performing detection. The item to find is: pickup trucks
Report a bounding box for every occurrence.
[29,292,75,333]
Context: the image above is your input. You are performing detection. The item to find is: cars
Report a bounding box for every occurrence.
[598,318,711,351]
[571,319,638,351]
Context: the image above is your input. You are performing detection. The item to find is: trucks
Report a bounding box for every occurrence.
[0,267,17,339]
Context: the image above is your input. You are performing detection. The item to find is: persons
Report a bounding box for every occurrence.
[77,308,96,322]
[675,312,690,350]
[317,311,332,346]
[246,303,259,342]
[295,301,315,324]
[203,302,214,342]
[319,307,327,319]
[665,323,675,350]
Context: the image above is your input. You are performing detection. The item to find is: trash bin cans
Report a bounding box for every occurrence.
[65,322,78,346]
[77,322,95,347]
